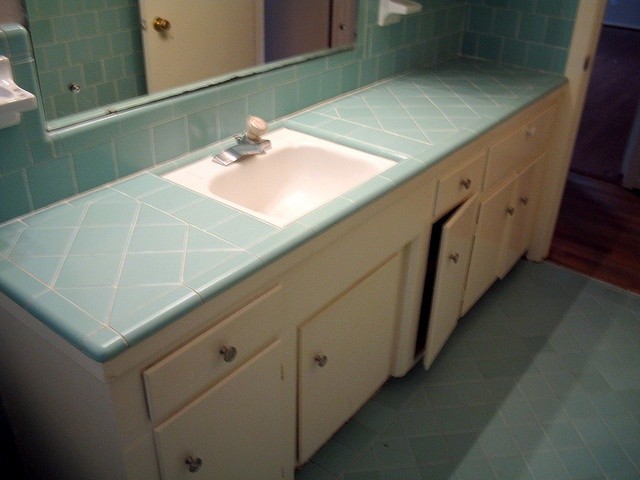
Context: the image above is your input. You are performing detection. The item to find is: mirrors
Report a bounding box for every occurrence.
[23,0,359,133]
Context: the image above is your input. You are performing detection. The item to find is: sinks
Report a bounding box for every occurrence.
[206,143,390,225]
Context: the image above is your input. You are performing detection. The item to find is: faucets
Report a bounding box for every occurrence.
[233,135,273,159]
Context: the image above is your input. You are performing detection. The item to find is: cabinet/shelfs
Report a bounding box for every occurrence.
[459,102,564,318]
[142,283,297,480]
[412,150,490,372]
[297,179,438,467]
